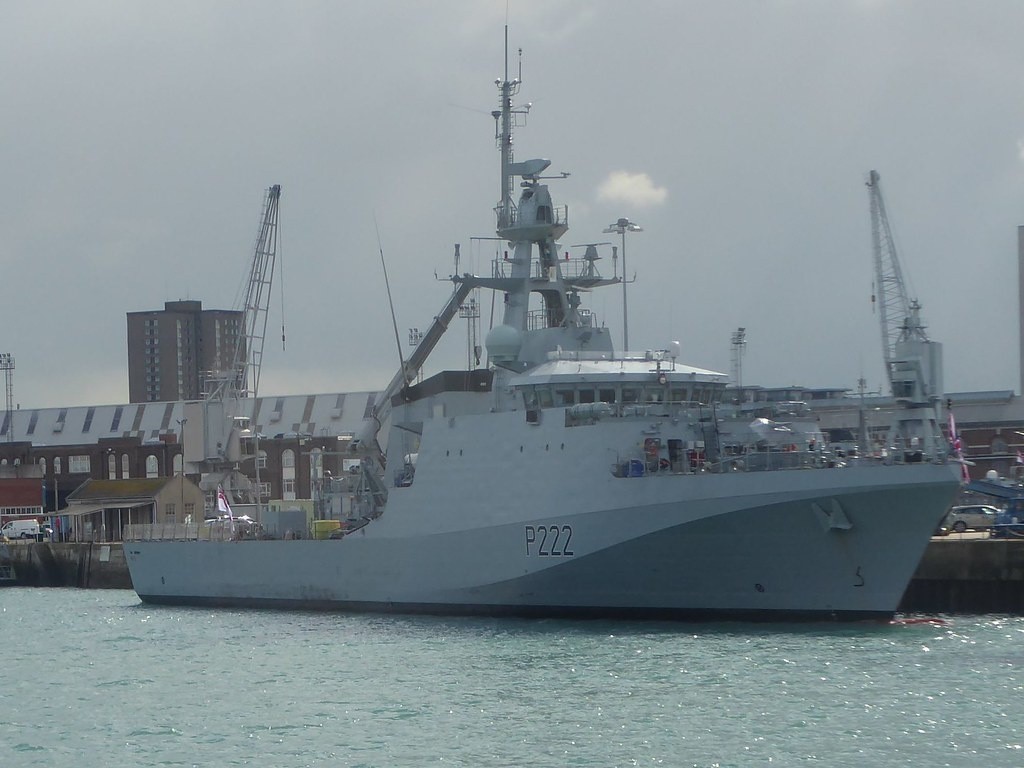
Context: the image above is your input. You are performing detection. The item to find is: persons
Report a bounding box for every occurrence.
[809,437,817,451]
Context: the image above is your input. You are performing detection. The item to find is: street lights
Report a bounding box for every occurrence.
[174,418,190,523]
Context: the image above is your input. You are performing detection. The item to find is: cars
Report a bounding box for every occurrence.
[944,504,1004,533]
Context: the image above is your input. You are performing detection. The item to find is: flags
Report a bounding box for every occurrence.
[218,484,235,532]
[947,412,971,482]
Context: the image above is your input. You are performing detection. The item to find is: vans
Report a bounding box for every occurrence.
[0,518,40,538]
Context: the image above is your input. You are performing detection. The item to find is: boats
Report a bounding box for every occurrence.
[121,23,964,628]
